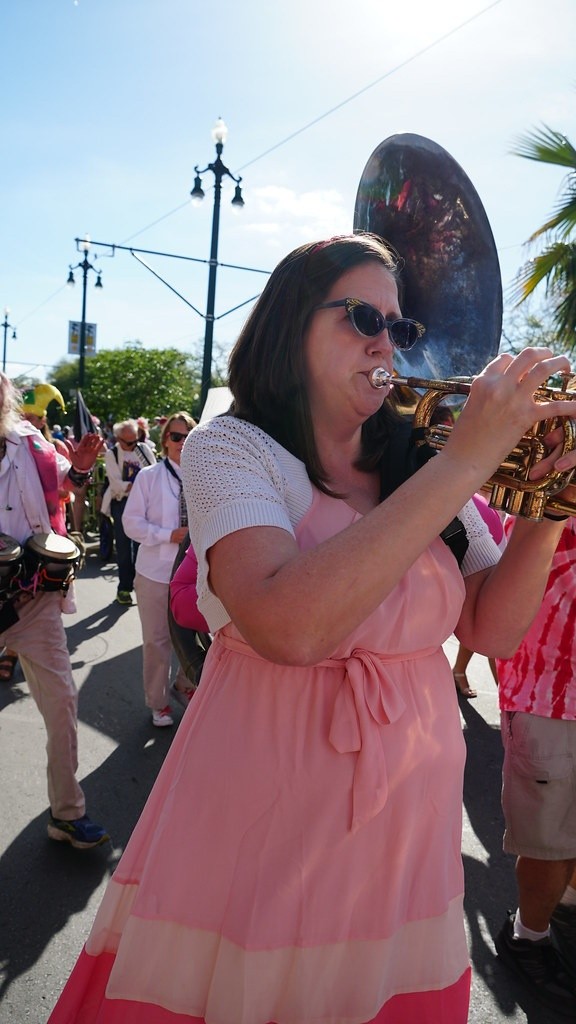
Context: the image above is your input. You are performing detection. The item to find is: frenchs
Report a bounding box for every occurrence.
[354,133,503,416]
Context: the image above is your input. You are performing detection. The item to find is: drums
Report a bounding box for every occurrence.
[1,534,25,590]
[26,533,81,592]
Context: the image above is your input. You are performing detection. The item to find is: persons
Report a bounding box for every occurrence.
[0,231,576,1021]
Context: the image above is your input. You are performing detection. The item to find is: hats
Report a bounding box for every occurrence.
[16,383,68,416]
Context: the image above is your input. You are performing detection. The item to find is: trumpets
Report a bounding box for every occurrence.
[369,370,576,523]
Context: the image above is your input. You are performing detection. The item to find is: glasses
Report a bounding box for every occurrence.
[117,437,139,446]
[169,431,188,442]
[313,297,425,352]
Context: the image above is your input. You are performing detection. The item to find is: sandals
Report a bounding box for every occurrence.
[454,670,477,698]
[0,650,18,680]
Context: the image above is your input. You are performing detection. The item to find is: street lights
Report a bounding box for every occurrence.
[0,313,19,373]
[190,131,245,427]
[68,247,104,392]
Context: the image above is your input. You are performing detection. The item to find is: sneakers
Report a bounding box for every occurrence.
[495,909,576,1016]
[152,705,174,726]
[171,680,198,709]
[47,807,110,850]
[550,903,576,975]
[116,588,132,604]
